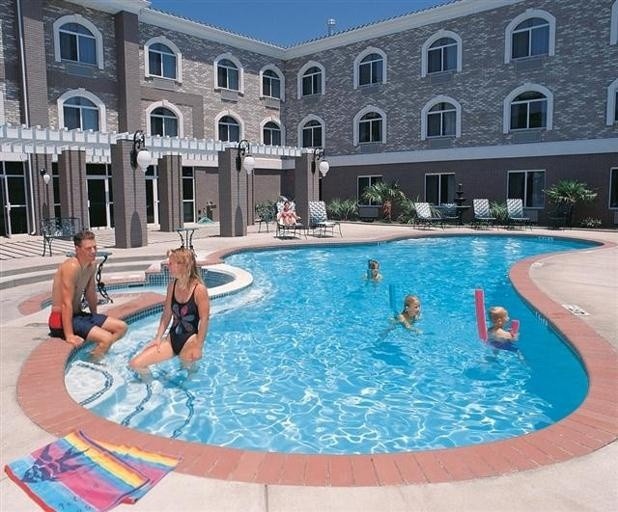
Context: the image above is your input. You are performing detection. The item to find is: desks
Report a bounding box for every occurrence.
[176,228,197,257]
[66,251,114,303]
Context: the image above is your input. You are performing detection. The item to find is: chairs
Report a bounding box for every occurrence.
[442,203,459,226]
[276,201,306,240]
[507,199,530,230]
[413,202,443,230]
[473,199,498,229]
[307,201,343,238]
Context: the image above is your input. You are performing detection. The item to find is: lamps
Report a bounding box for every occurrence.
[312,146,329,177]
[236,140,255,175]
[40,168,50,184]
[130,130,151,172]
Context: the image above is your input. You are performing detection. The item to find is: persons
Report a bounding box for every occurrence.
[50,231,128,361]
[279,202,297,227]
[364,260,382,280]
[395,294,420,337]
[487,307,518,344]
[130,250,209,367]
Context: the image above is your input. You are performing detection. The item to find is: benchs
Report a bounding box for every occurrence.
[40,217,87,257]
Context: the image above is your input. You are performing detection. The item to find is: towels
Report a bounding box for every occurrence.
[5,429,184,512]
